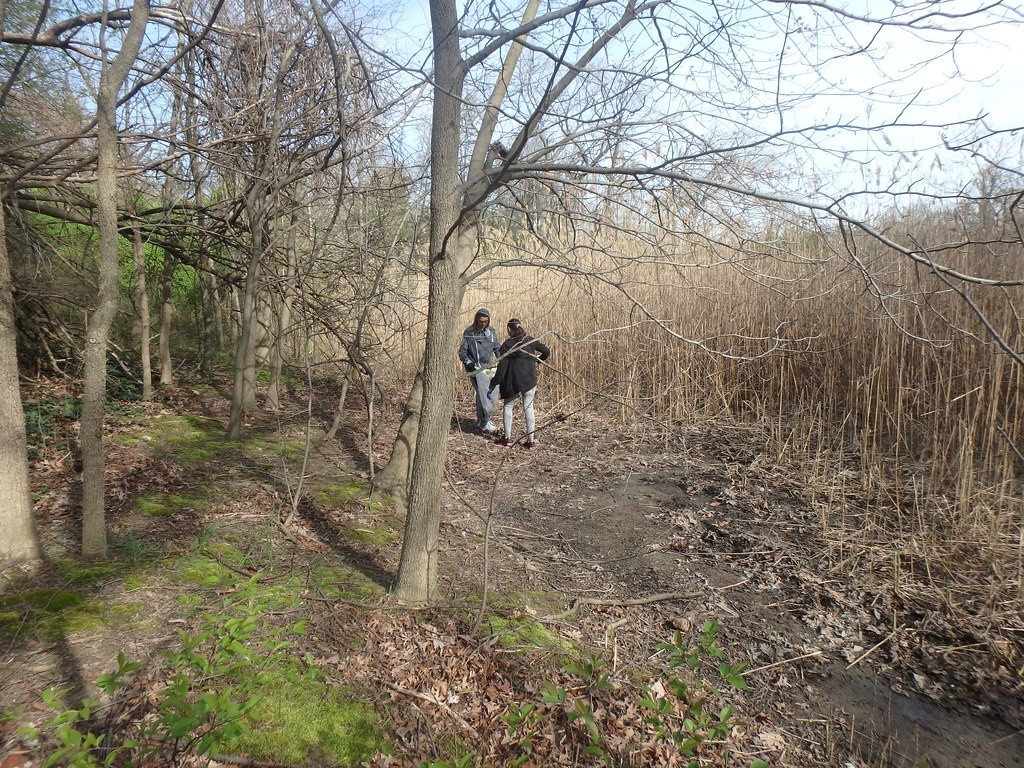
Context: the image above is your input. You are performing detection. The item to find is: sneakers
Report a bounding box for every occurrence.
[523,440,536,450]
[494,436,512,447]
[477,421,496,431]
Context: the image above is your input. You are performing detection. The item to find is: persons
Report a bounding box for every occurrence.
[487,318,550,451]
[459,308,502,431]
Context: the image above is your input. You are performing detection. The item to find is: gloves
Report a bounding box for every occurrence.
[468,363,476,372]
[487,389,493,400]
